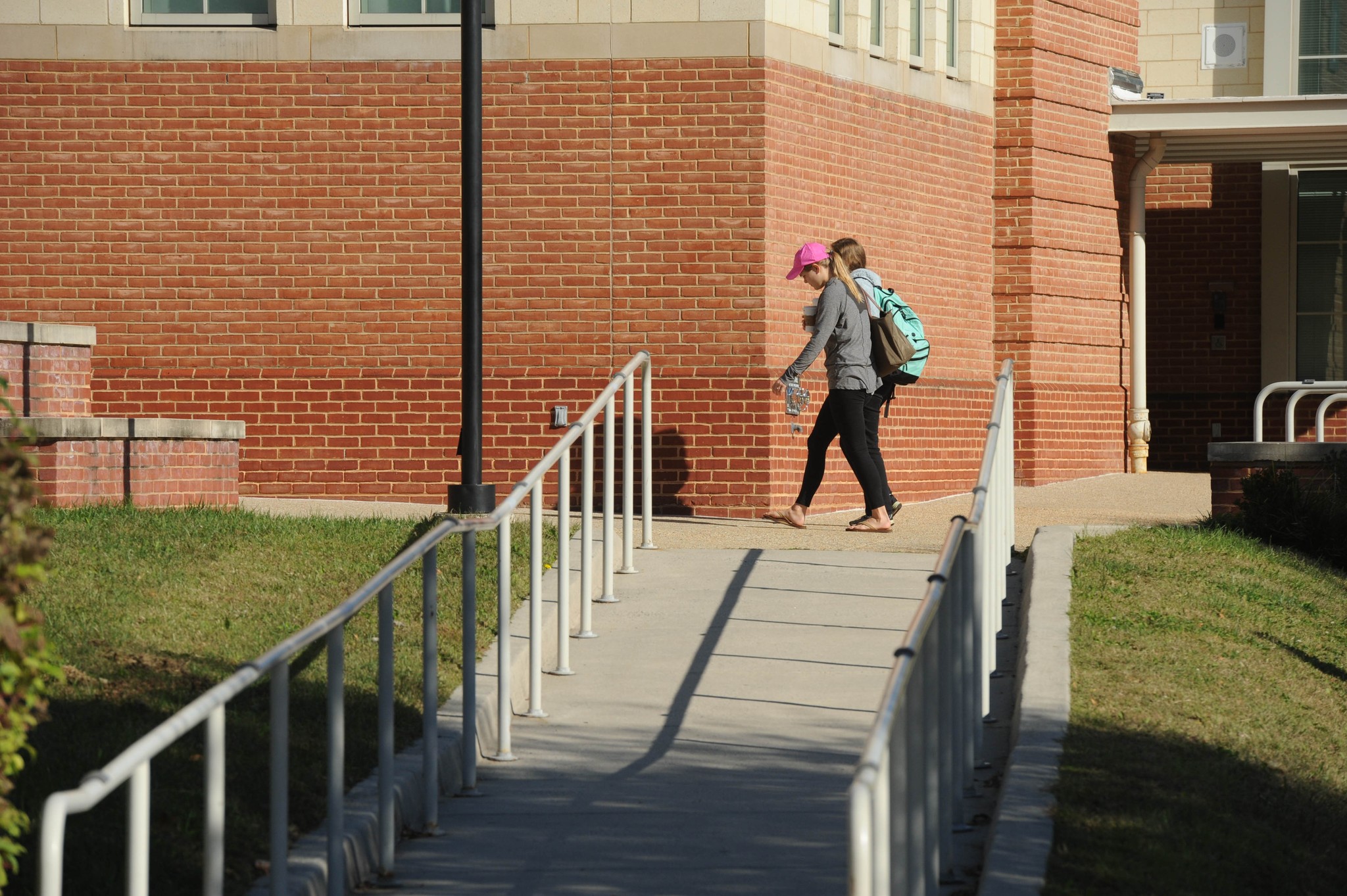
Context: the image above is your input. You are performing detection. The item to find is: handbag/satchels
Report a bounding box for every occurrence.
[854,282,916,377]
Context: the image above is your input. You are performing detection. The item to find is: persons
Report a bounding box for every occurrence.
[801,239,902,528]
[761,243,893,532]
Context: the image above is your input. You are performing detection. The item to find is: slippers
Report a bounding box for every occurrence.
[762,510,807,529]
[846,519,893,533]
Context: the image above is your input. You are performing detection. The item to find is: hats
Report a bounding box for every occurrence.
[784,243,831,279]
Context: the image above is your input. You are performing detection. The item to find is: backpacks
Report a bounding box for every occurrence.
[854,274,930,385]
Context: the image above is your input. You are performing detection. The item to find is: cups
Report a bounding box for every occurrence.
[804,306,817,332]
[812,298,819,306]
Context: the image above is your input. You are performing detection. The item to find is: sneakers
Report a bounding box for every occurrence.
[889,494,902,516]
[849,510,895,526]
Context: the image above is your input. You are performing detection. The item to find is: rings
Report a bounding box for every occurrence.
[777,387,780,390]
